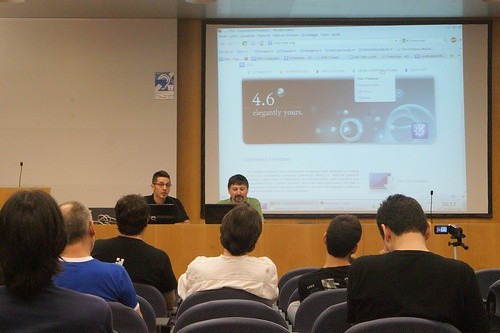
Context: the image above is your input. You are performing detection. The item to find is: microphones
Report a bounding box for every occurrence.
[430,190,433,224]
[19,162,24,187]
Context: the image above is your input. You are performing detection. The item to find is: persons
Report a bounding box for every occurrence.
[52,201,143,318]
[177,204,279,304]
[91,194,178,309]
[287,215,362,327]
[217,174,264,220]
[144,170,190,224]
[347,194,486,333]
[0,190,113,333]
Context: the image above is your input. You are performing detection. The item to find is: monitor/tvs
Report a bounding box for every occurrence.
[147,203,183,224]
[205,203,238,224]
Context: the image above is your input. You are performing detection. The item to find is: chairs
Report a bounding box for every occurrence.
[105,261,500,333]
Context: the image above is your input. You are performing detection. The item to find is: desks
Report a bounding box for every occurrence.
[90,223,500,282]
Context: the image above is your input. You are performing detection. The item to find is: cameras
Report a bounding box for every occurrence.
[435,223,466,240]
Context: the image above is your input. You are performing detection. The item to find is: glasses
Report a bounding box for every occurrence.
[154,182,172,186]
